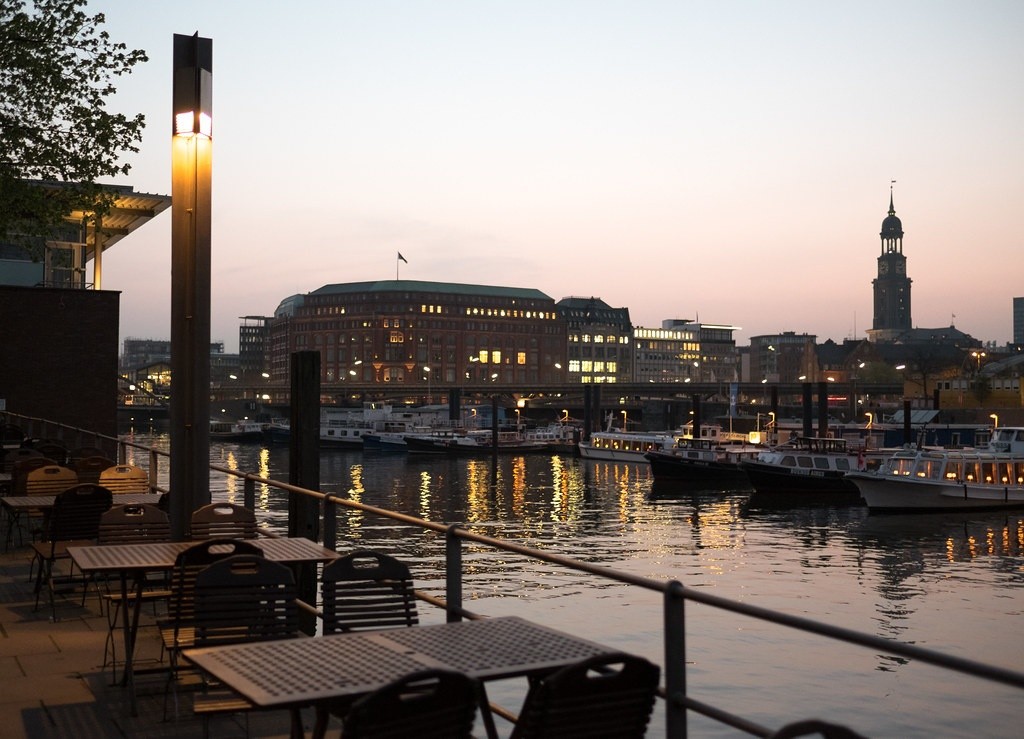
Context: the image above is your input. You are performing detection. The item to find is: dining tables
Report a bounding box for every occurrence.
[0,442,659,738]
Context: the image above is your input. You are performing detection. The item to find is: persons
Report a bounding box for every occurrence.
[876,403,885,425]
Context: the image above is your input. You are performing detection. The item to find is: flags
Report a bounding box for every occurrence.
[398,252,409,265]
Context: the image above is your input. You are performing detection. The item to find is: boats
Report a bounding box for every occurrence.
[404,429,569,452]
[578,432,672,462]
[641,437,767,490]
[320,416,433,442]
[209,418,291,441]
[841,450,1024,515]
[742,447,888,504]
